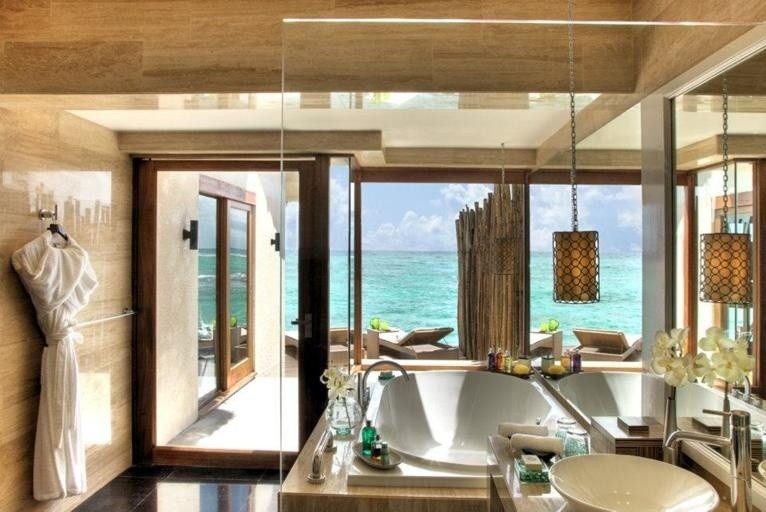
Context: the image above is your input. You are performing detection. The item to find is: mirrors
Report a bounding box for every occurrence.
[668,46,765,408]
[526,99,643,438]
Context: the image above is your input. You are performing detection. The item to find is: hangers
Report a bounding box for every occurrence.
[44,212,67,244]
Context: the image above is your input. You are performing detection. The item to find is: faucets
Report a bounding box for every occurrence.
[665,407,752,512]
[741,376,753,402]
[362,360,410,407]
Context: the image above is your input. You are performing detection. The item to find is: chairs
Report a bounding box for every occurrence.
[197,324,247,379]
[570,325,643,362]
[529,327,563,356]
[361,323,460,359]
[283,325,361,366]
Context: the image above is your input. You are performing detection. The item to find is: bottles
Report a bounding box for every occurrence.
[361,419,390,464]
[555,417,576,438]
[488,347,512,372]
[562,347,583,372]
[564,429,590,457]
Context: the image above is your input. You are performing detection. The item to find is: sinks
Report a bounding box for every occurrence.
[547,453,721,512]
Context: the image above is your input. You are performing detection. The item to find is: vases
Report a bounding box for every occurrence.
[663,395,680,456]
[721,397,731,460]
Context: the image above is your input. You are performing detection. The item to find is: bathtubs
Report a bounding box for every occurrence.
[530,362,766,440]
[359,366,590,488]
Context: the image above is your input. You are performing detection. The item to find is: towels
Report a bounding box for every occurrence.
[497,422,549,436]
[510,432,565,456]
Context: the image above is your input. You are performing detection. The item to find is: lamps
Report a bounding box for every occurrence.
[699,74,751,305]
[553,2,601,305]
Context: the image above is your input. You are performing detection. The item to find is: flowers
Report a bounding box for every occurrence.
[318,357,358,426]
[649,324,714,398]
[697,327,757,401]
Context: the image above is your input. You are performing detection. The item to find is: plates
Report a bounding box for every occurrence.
[353,442,403,469]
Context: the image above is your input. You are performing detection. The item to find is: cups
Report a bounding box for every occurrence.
[542,355,556,373]
